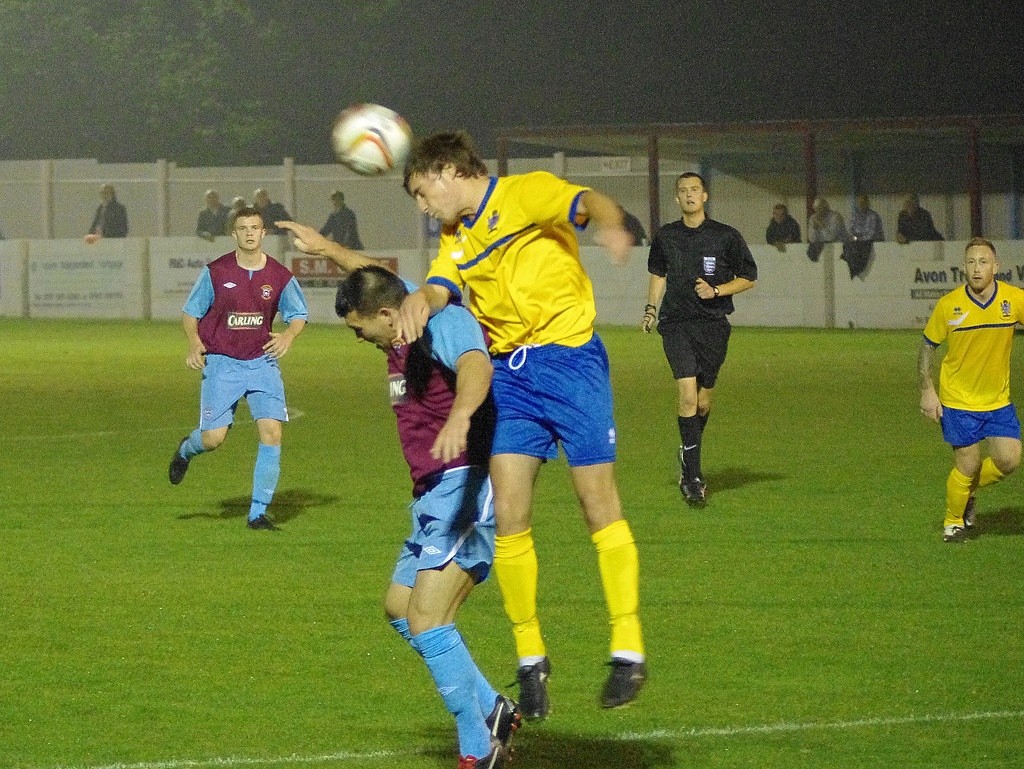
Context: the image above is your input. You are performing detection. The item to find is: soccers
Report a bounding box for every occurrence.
[331,103,416,177]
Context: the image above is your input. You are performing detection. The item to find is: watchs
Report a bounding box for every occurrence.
[712,285,720,299]
[644,303,657,313]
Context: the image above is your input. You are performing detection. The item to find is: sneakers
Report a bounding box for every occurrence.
[482,694,522,769]
[679,473,707,505]
[941,524,966,543]
[247,514,281,532]
[963,496,977,529]
[505,656,554,723]
[457,734,500,769]
[678,445,687,474]
[168,436,190,484]
[601,657,647,707]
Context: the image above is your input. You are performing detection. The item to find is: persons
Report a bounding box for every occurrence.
[640,170,759,504]
[270,216,520,769]
[849,194,886,242]
[195,189,230,243]
[614,205,649,247]
[83,183,127,243]
[917,236,1024,543]
[252,189,295,235]
[809,197,849,243]
[319,189,365,251]
[394,121,650,730]
[764,204,803,252]
[168,209,310,529]
[895,192,946,244]
[223,197,253,236]
